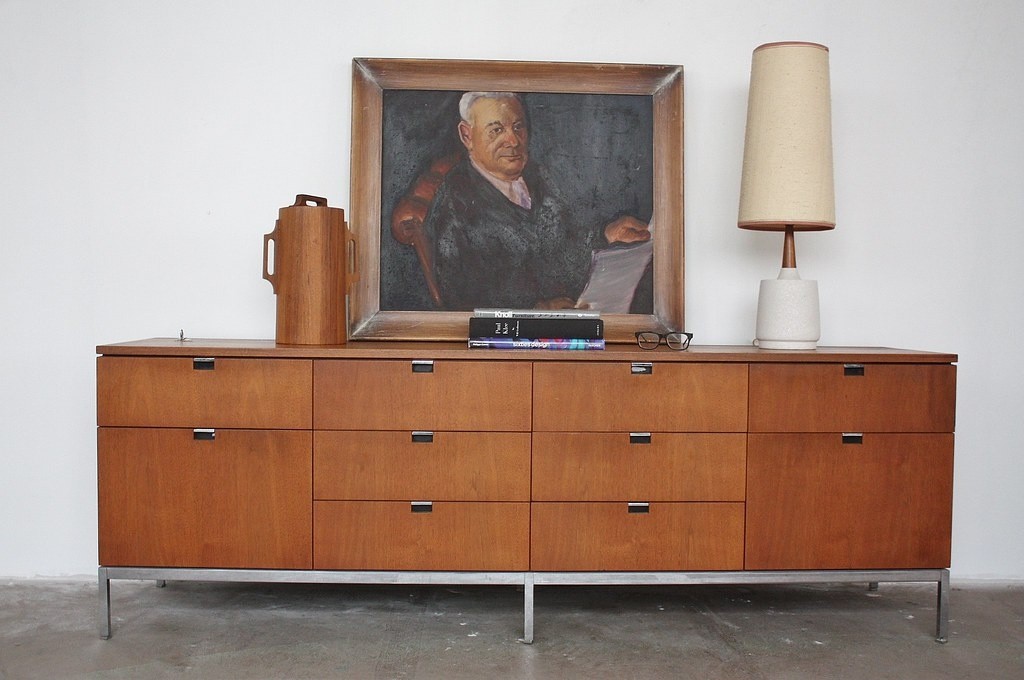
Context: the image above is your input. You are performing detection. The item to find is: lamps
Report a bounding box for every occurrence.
[738,40,836,350]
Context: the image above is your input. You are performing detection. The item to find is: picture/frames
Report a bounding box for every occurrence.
[346,55,685,344]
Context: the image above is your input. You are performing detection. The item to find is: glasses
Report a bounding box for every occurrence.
[635,331,693,350]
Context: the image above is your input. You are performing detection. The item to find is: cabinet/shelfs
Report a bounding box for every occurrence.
[95,334,960,644]
